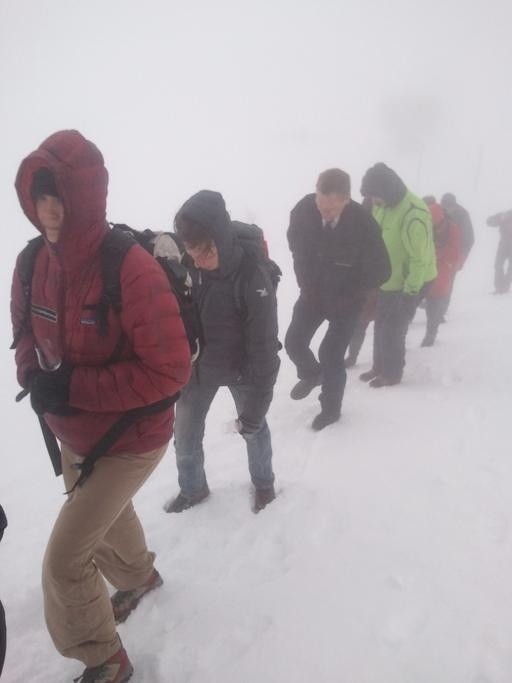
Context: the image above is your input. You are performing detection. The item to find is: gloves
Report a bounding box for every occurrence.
[235,398,270,442]
[26,367,69,413]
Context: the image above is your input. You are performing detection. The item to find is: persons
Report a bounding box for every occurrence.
[341,290,382,365]
[441,191,476,270]
[283,169,392,430]
[359,160,441,387]
[414,194,462,347]
[484,209,512,294]
[161,189,284,515]
[8,128,191,682]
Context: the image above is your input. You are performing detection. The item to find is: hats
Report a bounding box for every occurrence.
[31,167,55,199]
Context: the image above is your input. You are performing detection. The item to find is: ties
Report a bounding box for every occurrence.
[324,221,333,232]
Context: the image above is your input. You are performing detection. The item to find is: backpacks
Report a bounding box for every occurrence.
[18,226,204,368]
[229,219,281,313]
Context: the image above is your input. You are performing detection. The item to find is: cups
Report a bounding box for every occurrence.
[223,419,243,435]
[33,339,62,372]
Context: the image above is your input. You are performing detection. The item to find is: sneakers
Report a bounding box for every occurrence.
[73,647,135,682]
[111,567,164,625]
[359,368,379,382]
[166,488,210,514]
[291,364,323,401]
[312,409,341,431]
[255,482,275,514]
[421,333,435,348]
[369,375,401,388]
[344,357,356,369]
